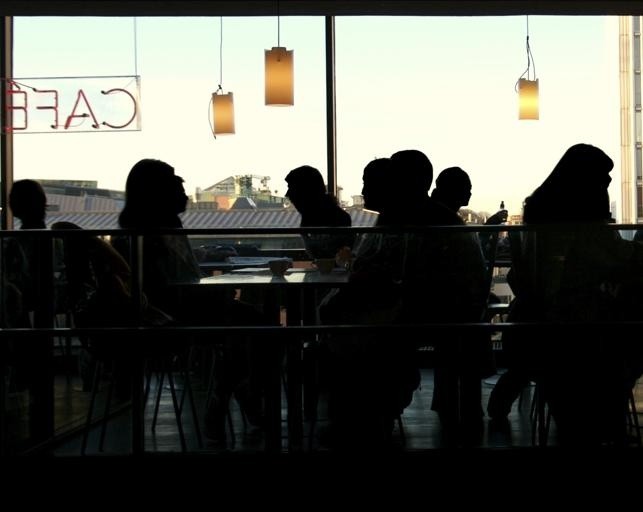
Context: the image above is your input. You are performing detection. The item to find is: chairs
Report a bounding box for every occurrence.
[0,224,643,458]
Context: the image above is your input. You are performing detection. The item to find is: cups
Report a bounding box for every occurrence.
[269,259,289,276]
[311,258,334,274]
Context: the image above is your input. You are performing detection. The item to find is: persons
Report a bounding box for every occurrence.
[486,265,536,431]
[4,181,64,315]
[431,168,507,433]
[524,144,642,455]
[315,150,441,446]
[117,160,281,448]
[284,166,351,262]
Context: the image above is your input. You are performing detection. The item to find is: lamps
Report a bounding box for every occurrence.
[263,16,295,108]
[514,15,540,121]
[207,16,236,137]
